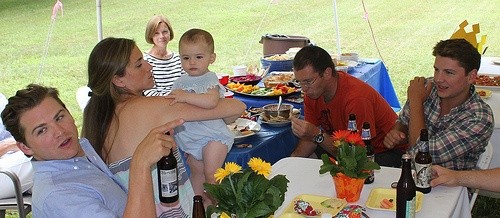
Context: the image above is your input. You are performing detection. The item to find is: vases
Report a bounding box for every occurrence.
[333,174,364,201]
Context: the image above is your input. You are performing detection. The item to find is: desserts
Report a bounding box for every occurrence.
[293,200,317,216]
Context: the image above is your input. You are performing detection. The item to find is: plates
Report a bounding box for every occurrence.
[231,117,261,143]
[259,117,291,127]
[365,188,423,212]
[281,194,347,218]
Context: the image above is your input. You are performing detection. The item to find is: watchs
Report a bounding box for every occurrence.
[312,128,325,144]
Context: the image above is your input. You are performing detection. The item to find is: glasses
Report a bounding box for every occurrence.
[293,69,325,87]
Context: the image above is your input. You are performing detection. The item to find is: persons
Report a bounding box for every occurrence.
[0,92,34,197]
[165,29,235,211]
[80,37,247,218]
[413,164,500,193]
[0,83,177,217]
[290,45,409,168]
[143,15,187,97]
[382,39,495,201]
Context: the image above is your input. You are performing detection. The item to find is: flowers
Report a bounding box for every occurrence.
[203,157,292,218]
[319,128,381,178]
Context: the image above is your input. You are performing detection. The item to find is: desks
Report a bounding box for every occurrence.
[473,55,500,198]
[179,58,402,174]
[266,157,473,218]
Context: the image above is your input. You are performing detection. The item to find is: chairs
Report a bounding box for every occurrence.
[467,139,494,209]
[0,168,33,218]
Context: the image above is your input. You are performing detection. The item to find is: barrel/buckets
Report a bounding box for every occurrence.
[262,34,308,55]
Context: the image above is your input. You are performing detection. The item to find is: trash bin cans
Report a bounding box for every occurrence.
[259,34,311,61]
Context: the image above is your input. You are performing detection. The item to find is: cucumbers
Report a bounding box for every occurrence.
[321,199,343,209]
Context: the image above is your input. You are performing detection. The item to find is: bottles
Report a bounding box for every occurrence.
[348,114,357,133]
[157,130,179,202]
[360,122,375,184]
[192,196,206,218]
[416,129,432,193]
[396,154,416,218]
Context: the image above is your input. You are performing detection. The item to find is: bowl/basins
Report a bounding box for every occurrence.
[263,104,293,119]
[262,54,293,71]
[231,76,262,86]
[335,66,347,73]
[340,53,358,62]
[234,60,271,76]
